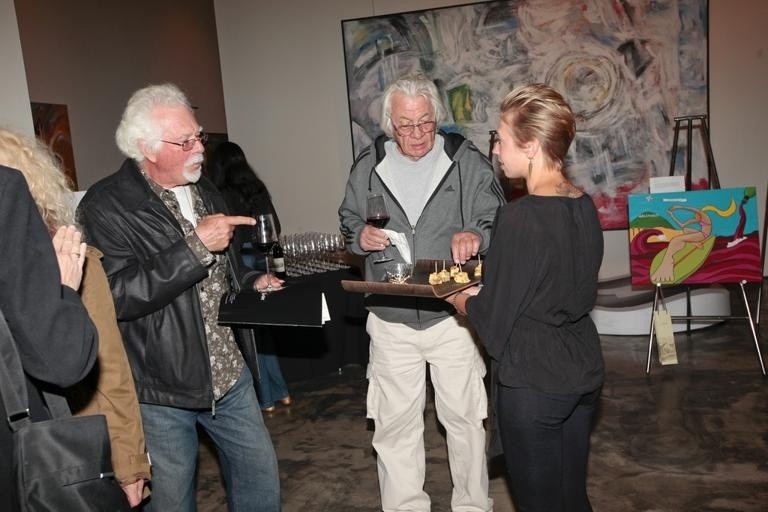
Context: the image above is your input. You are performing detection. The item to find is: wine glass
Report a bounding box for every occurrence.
[250,212,281,294]
[361,194,398,266]
[281,231,349,275]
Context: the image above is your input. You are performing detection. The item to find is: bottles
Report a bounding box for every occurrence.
[272,241,287,279]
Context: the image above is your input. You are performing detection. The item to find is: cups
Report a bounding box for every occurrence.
[387,264,412,286]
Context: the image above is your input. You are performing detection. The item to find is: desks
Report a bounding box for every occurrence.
[260,257,367,384]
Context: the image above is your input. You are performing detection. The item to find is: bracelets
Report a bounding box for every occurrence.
[453,292,462,314]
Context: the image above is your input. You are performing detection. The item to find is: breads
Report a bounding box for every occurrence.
[474,264,482,275]
[450,266,459,277]
[429,272,442,285]
[438,270,450,282]
[454,272,470,283]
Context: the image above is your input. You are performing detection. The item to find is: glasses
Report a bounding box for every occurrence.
[393,121,436,137]
[162,131,204,151]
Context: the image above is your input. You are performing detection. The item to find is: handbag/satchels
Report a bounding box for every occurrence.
[10,414,131,512]
[654,284,679,365]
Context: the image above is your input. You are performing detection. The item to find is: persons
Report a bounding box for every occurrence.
[340,76,508,512]
[446,84,604,512]
[206,140,292,410]
[1,131,154,512]
[76,86,283,511]
